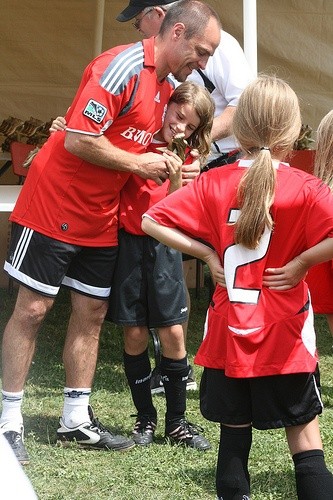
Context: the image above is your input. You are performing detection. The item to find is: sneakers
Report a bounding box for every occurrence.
[56,404,136,452]
[165,413,212,451]
[0,421,31,465]
[130,411,158,446]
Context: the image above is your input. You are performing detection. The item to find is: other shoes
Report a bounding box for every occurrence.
[147,368,198,393]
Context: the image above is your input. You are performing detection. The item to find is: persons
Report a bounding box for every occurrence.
[117,0,251,396]
[141,75,333,500]
[48,81,216,450]
[305,109,333,399]
[0,0,220,464]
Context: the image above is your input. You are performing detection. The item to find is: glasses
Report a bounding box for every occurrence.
[132,6,167,29]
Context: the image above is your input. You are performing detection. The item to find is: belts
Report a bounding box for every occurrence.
[201,148,243,172]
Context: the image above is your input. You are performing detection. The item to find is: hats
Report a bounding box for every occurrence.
[115,0,179,22]
